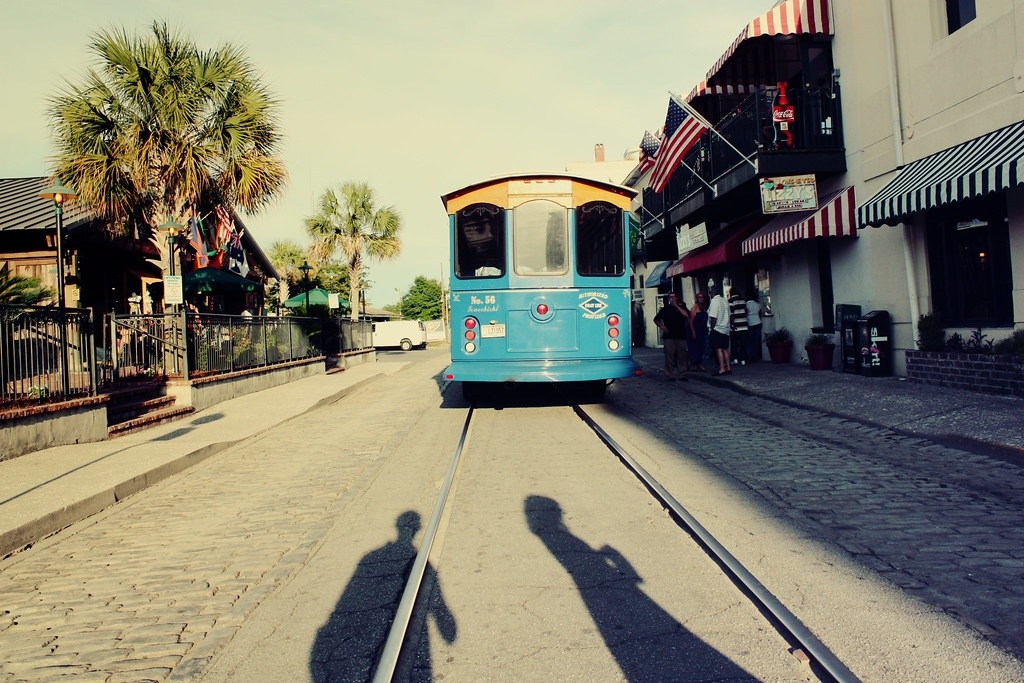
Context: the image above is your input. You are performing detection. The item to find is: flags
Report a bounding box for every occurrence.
[647,96,709,195]
[638,129,661,175]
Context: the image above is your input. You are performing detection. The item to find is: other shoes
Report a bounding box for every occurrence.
[680,377,688,381]
[689,364,705,371]
[730,359,738,366]
[712,370,726,376]
[739,360,745,366]
[670,377,675,382]
[726,370,732,374]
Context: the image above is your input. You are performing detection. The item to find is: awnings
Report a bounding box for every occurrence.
[854,119,1024,230]
[644,185,859,289]
[661,0,835,145]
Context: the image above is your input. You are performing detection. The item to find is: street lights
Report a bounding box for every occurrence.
[36,178,78,401]
[299,260,314,318]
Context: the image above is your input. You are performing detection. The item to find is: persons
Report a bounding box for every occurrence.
[653,285,762,376]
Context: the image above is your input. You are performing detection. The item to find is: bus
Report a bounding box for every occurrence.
[440,172,646,398]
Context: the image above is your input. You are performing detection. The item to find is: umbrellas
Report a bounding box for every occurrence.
[284,286,350,309]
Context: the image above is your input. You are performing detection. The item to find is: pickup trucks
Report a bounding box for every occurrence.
[342,314,430,351]
